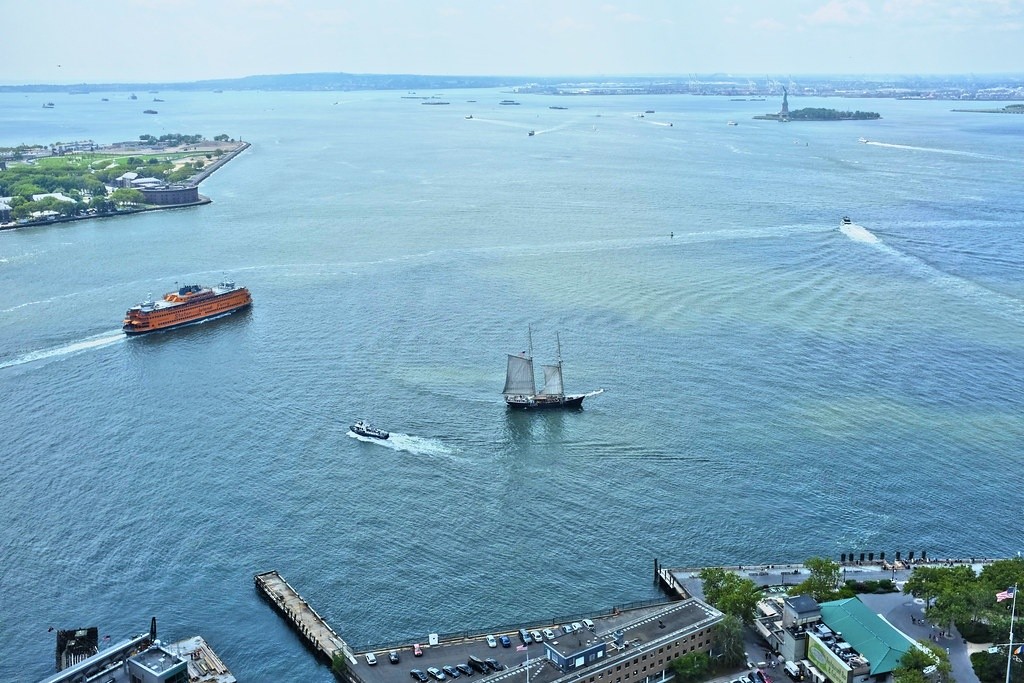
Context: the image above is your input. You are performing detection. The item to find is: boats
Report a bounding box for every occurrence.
[528,130,534,135]
[857,136,868,143]
[122,271,252,336]
[465,114,473,119]
[349,421,390,439]
[842,215,850,224]
[727,121,739,125]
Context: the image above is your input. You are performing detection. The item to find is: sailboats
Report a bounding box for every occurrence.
[500,323,586,413]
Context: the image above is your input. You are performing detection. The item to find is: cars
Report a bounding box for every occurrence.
[730,679,741,683]
[364,618,596,683]
[738,675,753,683]
[748,672,763,683]
[758,670,774,683]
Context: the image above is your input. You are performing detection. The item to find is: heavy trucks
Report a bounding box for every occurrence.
[784,661,800,678]
